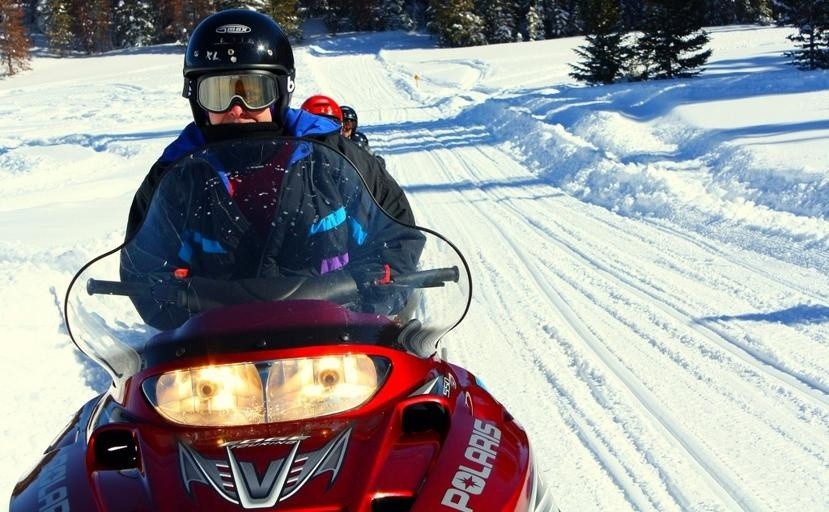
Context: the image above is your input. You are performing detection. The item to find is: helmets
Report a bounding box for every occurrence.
[302,96,344,124]
[183,9,295,124]
[339,105,356,131]
[352,132,368,147]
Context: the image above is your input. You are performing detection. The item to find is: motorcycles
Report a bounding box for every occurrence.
[8,138,560,512]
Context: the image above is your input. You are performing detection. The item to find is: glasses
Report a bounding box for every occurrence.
[182,68,295,112]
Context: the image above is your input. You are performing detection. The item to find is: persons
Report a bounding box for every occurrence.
[353,132,386,169]
[339,106,358,138]
[301,95,343,134]
[119,8,425,329]
